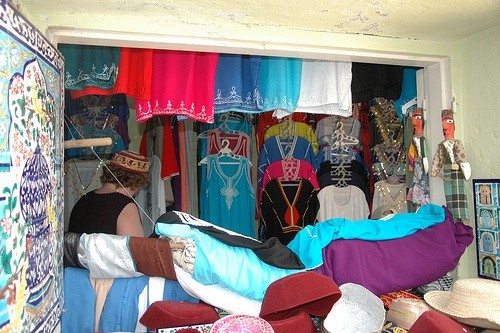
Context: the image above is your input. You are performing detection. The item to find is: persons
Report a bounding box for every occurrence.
[67,149,149,238]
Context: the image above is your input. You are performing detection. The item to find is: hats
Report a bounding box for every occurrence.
[210,313,275,333]
[261,309,318,333]
[322,282,386,332]
[139,301,221,331]
[110,150,150,174]
[259,271,342,317]
[424,278,500,333]
[407,309,476,333]
[386,297,432,331]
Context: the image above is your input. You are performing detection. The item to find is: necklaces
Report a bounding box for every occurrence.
[101,185,118,192]
